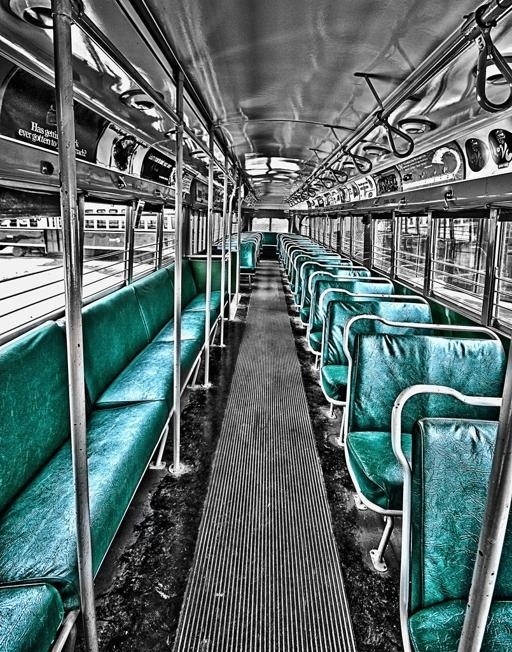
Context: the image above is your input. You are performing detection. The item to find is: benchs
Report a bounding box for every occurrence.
[261,230,512,652]
[1,233,262,651]
